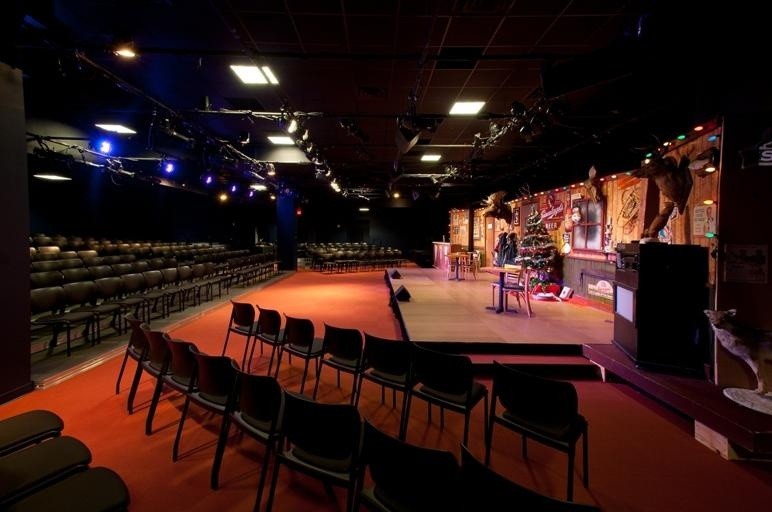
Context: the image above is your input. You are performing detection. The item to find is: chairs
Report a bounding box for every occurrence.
[446,251,480,280]
[300,242,405,271]
[0,405,129,511]
[21,239,281,358]
[503,270,536,317]
[491,266,522,310]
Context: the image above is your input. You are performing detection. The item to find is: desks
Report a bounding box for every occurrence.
[482,266,521,313]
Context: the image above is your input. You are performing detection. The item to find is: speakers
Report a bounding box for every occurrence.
[394,285,411,301]
[391,269,401,280]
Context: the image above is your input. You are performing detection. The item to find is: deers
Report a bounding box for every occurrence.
[631,135,692,215]
[480,191,512,224]
